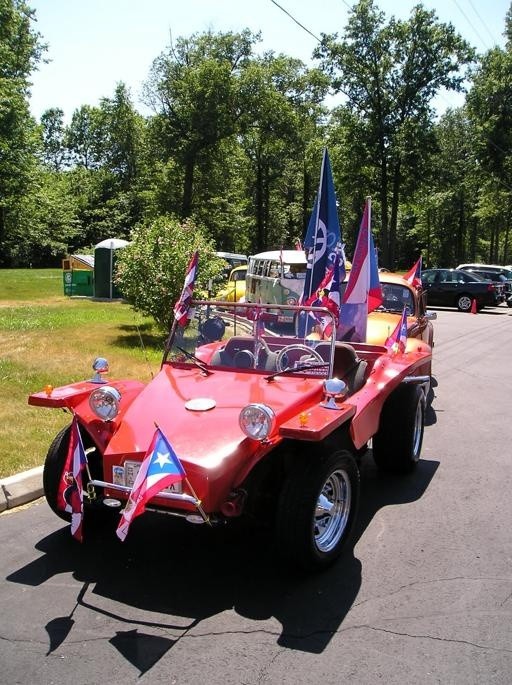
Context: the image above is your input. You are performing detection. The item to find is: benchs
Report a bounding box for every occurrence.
[253,335,387,381]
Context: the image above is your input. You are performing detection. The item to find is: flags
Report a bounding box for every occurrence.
[172,250,197,329]
[254,148,423,357]
[114,426,191,542]
[57,416,88,544]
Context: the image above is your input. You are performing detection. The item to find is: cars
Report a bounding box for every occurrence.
[211,251,249,313]
[304,271,435,377]
[420,264,512,312]
[27,299,439,570]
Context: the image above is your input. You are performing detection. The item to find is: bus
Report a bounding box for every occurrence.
[245,250,310,330]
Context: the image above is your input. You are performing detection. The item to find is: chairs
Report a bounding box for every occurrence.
[439,273,463,283]
[210,336,368,397]
